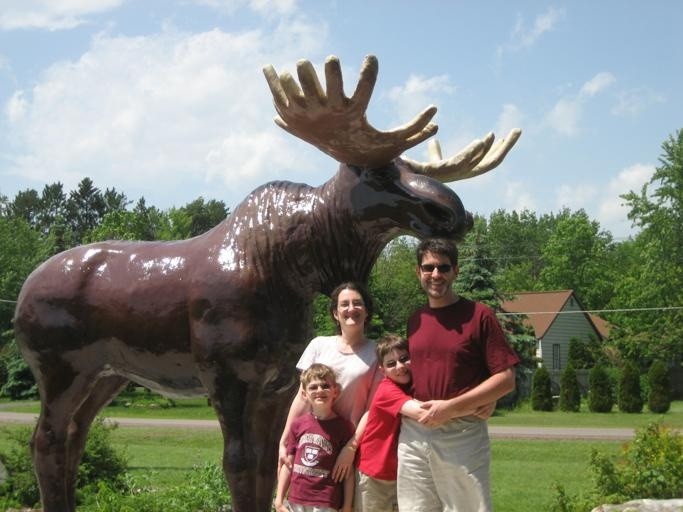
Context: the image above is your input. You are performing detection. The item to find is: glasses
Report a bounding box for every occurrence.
[386,354,409,368]
[308,382,332,389]
[420,264,453,273]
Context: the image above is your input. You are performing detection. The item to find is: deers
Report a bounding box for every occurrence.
[11,54,522,512]
